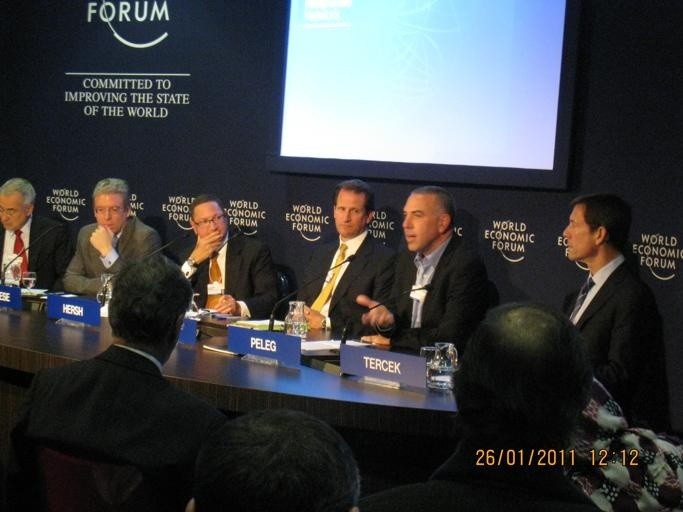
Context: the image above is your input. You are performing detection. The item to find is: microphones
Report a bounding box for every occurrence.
[1,222,68,285]
[269,254,357,336]
[101,230,189,307]
[188,231,244,321]
[341,284,434,346]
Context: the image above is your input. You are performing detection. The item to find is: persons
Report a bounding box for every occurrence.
[0,177,68,295]
[557,189,672,434]
[293,179,397,337]
[4,256,233,509]
[356,299,601,508]
[62,177,168,304]
[162,195,286,323]
[355,184,493,353]
[183,406,364,511]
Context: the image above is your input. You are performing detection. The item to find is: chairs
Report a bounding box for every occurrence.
[268,267,299,321]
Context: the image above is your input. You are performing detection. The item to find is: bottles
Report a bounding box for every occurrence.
[284,301,307,338]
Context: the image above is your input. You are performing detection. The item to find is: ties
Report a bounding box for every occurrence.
[568,277,594,322]
[310,244,347,312]
[204,251,223,309]
[112,235,117,249]
[13,229,27,286]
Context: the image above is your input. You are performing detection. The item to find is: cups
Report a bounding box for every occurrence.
[207,285,222,311]
[419,342,459,394]
[5,256,20,285]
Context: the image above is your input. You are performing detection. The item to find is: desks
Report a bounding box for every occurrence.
[0,297,465,479]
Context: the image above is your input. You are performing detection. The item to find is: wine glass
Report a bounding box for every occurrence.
[100,273,113,301]
[21,271,35,295]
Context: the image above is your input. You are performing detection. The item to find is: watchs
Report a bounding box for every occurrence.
[321,316,327,328]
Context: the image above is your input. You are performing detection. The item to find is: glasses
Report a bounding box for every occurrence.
[93,206,119,214]
[194,214,224,226]
[0,208,14,215]
